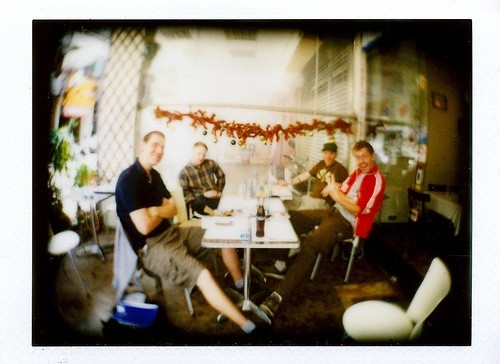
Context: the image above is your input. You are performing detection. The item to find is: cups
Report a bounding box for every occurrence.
[240,228,251,238]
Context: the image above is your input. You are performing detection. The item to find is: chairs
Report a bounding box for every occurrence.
[310,195,388,283]
[342,255,453,343]
[107,210,195,315]
[406,187,451,252]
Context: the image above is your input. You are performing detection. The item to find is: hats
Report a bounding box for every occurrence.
[321,142,337,152]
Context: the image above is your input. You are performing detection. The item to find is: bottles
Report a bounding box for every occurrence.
[256,204,266,237]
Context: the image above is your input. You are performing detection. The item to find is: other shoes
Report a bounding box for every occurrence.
[234,284,244,295]
[262,266,286,280]
[257,293,281,319]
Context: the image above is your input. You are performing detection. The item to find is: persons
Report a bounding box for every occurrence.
[279,142,348,210]
[115,131,258,337]
[259,141,384,316]
[178,142,225,215]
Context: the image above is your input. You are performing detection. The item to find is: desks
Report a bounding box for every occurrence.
[72,187,113,263]
[421,190,465,236]
[201,198,300,326]
